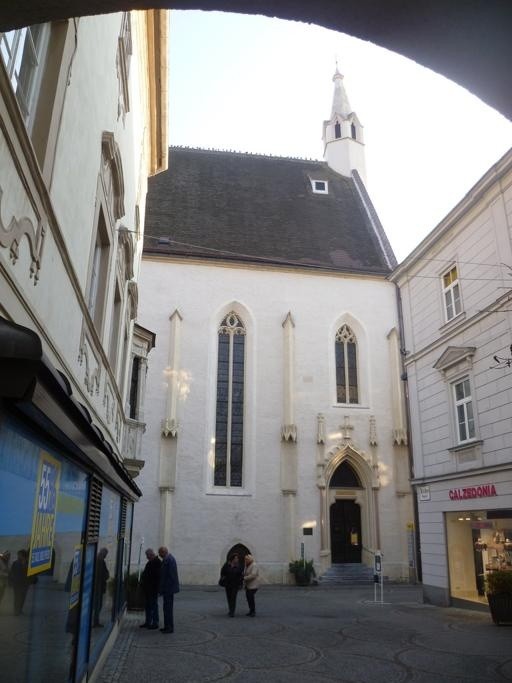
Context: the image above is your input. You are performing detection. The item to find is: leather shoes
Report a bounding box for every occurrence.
[139,623,174,632]
[246,611,257,618]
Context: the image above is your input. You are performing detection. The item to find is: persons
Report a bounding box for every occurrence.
[219,553,243,618]
[139,548,162,629]
[241,554,261,617]
[86,547,110,627]
[8,549,38,616]
[0,550,11,600]
[154,547,180,633]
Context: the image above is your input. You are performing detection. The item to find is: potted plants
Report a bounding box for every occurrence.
[484,572,512,626]
[287,558,318,587]
